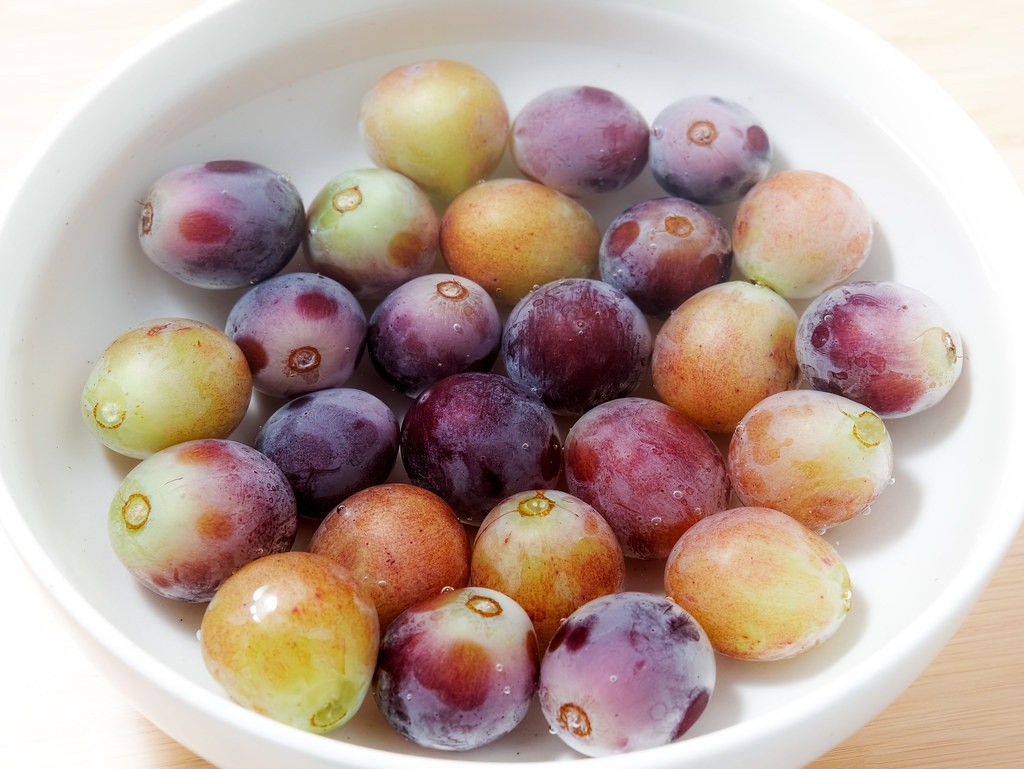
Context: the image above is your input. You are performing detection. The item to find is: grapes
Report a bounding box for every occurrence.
[85,60,965,758]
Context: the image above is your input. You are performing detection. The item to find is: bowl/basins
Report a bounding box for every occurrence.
[2,0,1024,769]
